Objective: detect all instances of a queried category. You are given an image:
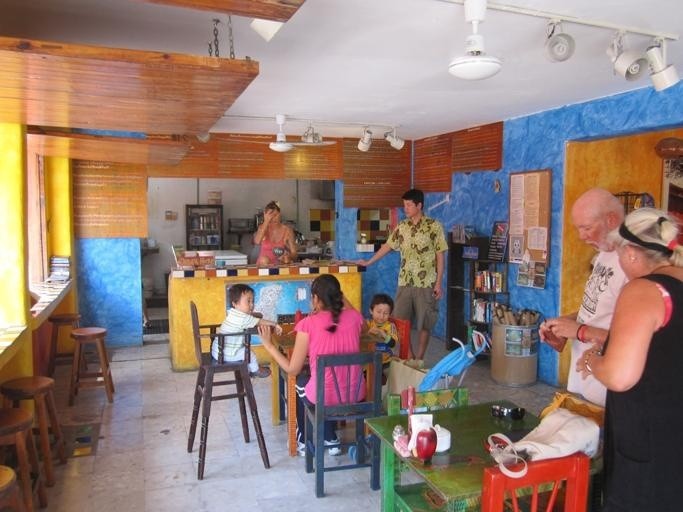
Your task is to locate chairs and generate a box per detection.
[183,298,274,482]
[387,387,472,512]
[277,313,307,421]
[170,244,184,268]
[302,350,384,498]
[481,450,590,512]
[381,313,410,359]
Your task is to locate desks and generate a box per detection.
[192,249,248,266]
[264,320,387,458]
[360,394,607,512]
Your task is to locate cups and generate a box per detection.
[216,261,225,268]
[489,405,525,420]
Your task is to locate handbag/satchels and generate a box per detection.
[541,389,605,428]
[487,407,601,477]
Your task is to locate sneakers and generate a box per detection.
[288,441,306,456]
[324,435,343,455]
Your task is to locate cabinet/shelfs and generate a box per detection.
[184,202,224,250]
[474,258,508,357]
[444,232,487,361]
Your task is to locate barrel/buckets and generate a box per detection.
[490,318,540,389]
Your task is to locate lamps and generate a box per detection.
[603,30,643,79]
[355,125,378,152]
[643,33,679,92]
[382,126,408,150]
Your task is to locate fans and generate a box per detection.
[438,0,500,81]
[220,114,337,153]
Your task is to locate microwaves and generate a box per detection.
[229,218,254,233]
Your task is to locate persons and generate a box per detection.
[355,188,449,360]
[286,219,305,246]
[364,293,400,378]
[211,283,282,378]
[253,201,297,266]
[257,273,365,457]
[538,188,628,408]
[576,207,683,512]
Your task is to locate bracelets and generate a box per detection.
[583,348,602,375]
[577,323,586,342]
[580,325,588,344]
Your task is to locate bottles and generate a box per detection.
[208,190,222,204]
[361,233,367,244]
[255,207,262,215]
[392,424,451,464]
[475,271,504,293]
[374,238,386,252]
[273,247,290,265]
[190,211,218,245]
[284,220,333,257]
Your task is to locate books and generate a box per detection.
[46,254,72,284]
[452,223,475,244]
[472,271,505,354]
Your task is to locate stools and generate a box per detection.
[0,375,68,487]
[0,464,24,512]
[66,327,115,406]
[0,407,50,511]
[43,312,91,380]
